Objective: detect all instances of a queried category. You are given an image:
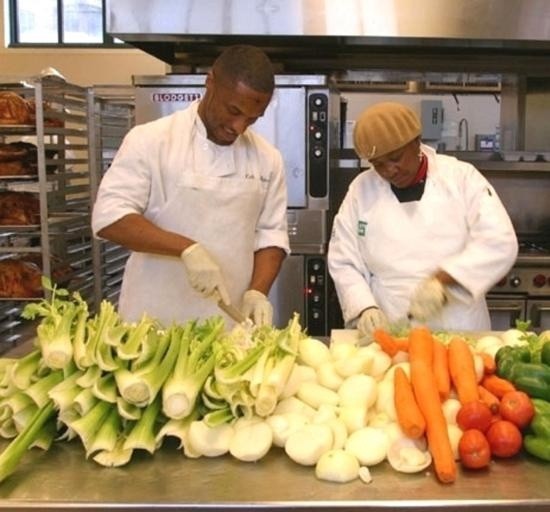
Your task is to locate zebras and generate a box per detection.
[129,73,343,335]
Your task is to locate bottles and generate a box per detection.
[352,102,423,161]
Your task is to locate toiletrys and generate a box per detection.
[495,342,550,461]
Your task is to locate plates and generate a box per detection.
[0,80,135,348]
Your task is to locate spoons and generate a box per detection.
[456,392,535,469]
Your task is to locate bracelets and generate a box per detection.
[447,150,550,161]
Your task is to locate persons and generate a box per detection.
[326,101,519,337]
[90,45,293,329]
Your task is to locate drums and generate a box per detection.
[210,289,246,324]
[354,294,447,348]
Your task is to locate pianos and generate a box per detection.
[372,327,516,485]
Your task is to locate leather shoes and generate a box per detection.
[240,288,275,333]
[408,278,450,323]
[356,302,392,342]
[180,243,230,310]
